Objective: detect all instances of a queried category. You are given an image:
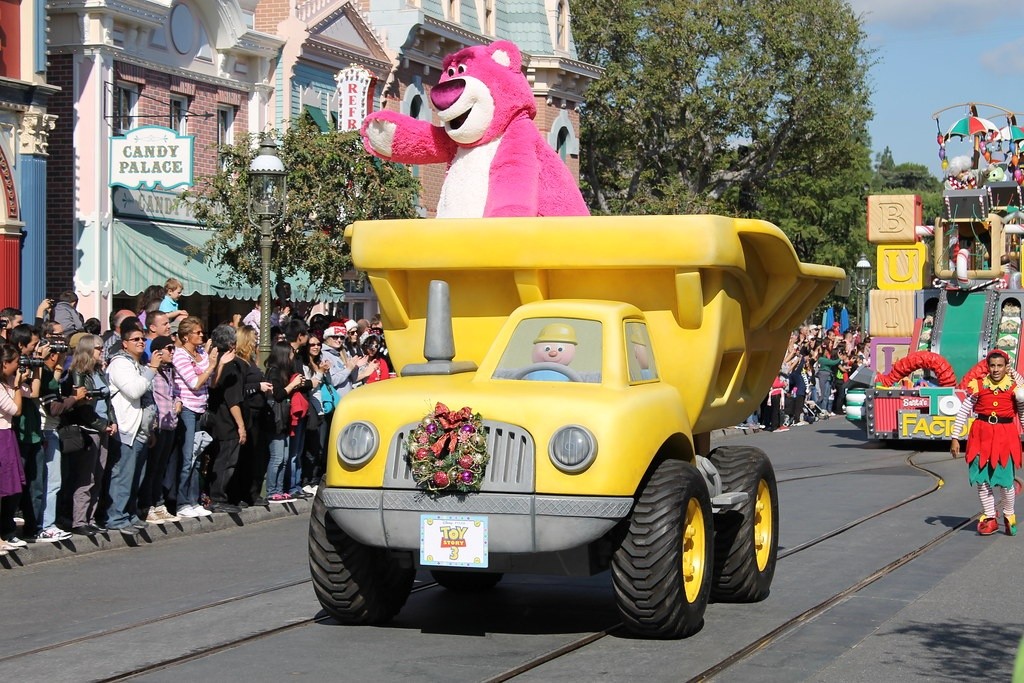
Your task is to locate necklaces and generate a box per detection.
[182,345,197,358]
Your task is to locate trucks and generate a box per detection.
[307,213,845,641]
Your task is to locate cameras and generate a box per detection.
[39,340,68,353]
[158,350,163,356]
[48,301,57,308]
[83,388,101,398]
[18,355,45,373]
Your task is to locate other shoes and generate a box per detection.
[0,541,19,556]
[980,518,999,535]
[1003,514,1017,536]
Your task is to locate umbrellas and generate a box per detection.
[998,125,1024,142]
[943,117,999,142]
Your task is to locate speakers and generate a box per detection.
[867,195,930,381]
[942,181,1019,219]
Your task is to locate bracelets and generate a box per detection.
[150,365,159,368]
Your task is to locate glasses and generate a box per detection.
[163,345,175,352]
[309,343,321,347]
[348,330,358,335]
[94,346,103,351]
[365,344,379,348]
[328,335,345,341]
[125,337,147,341]
[189,330,204,335]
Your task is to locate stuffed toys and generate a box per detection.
[983,162,1009,182]
[361,39,591,218]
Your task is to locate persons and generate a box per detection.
[733,322,872,433]
[135,285,165,329]
[142,311,277,523]
[0,298,164,556]
[44,291,83,336]
[243,294,398,503]
[951,349,1024,535]
[159,278,189,318]
[942,156,991,267]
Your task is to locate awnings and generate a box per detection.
[114,224,348,304]
[304,104,333,132]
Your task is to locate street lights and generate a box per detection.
[852,252,874,342]
[243,133,291,375]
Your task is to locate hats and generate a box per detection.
[341,317,358,332]
[150,336,176,354]
[359,331,380,350]
[323,319,347,340]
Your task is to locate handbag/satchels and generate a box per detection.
[320,376,341,415]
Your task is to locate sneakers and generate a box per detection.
[735,422,750,430]
[5,536,28,547]
[46,526,73,540]
[772,425,790,433]
[73,482,318,537]
[22,529,59,543]
